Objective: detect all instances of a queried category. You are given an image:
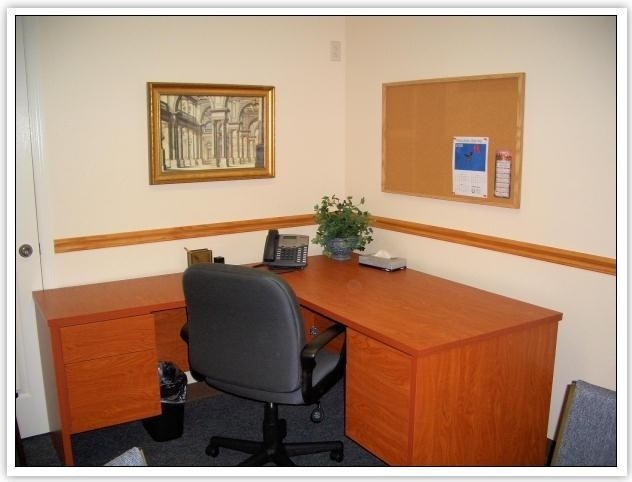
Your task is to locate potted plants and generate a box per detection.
[310,192,376,260]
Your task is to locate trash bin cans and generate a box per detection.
[141,361,187,443]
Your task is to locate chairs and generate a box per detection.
[179,261,346,468]
[550,379,617,467]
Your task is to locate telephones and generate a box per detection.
[263,229,309,270]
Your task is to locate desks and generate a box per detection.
[31,252,563,467]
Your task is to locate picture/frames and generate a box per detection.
[148,81,275,185]
